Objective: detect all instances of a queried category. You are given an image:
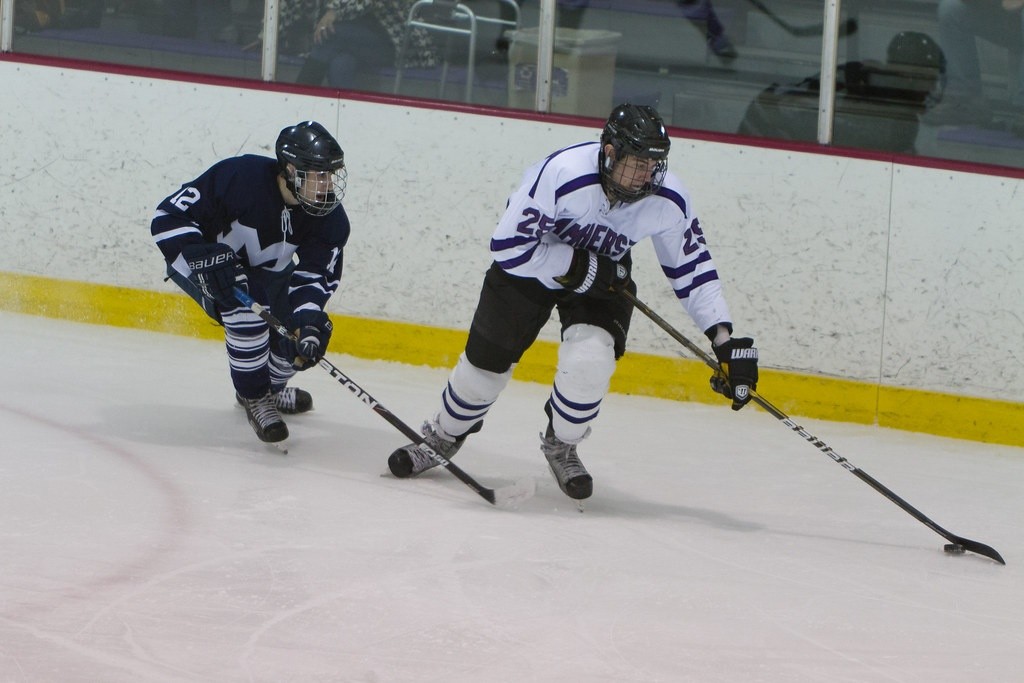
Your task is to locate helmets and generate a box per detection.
[275,121,347,216]
[599,103,671,203]
[886,31,947,72]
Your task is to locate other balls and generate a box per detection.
[943,543,966,554]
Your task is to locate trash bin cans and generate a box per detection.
[504,26,623,122]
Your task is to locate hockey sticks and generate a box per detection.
[234,282,558,513]
[620,283,1005,565]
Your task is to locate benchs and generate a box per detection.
[11,0,1024,168]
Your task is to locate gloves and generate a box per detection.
[184,244,248,308]
[710,337,759,411]
[280,309,333,371]
[552,248,630,301]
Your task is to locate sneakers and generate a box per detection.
[234,386,314,417]
[706,35,737,63]
[242,391,289,454]
[388,411,483,479]
[926,87,992,124]
[539,427,593,513]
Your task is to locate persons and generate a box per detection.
[12,0,438,93]
[147,119,351,444]
[391,104,761,499]
[546,1,949,152]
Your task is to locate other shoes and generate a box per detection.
[216,25,240,44]
